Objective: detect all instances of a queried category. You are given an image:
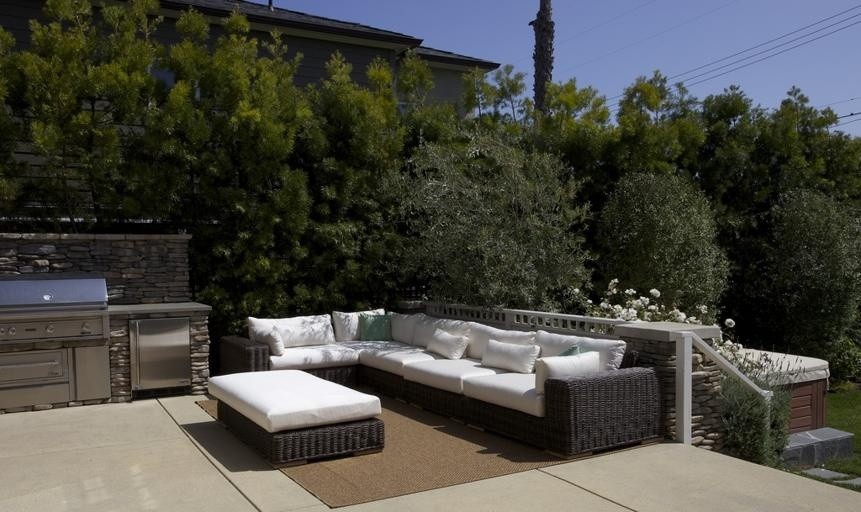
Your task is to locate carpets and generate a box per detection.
[194,384,665,509]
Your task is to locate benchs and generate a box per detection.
[206,369,385,468]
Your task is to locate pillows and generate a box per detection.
[579,335,627,371]
[413,314,470,357]
[248,327,286,357]
[533,350,602,395]
[531,331,581,376]
[332,308,385,342]
[385,311,425,347]
[359,314,392,342]
[426,327,470,360]
[479,338,540,375]
[467,321,536,363]
[558,344,581,357]
[246,313,337,348]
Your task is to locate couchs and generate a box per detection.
[220,308,666,460]
